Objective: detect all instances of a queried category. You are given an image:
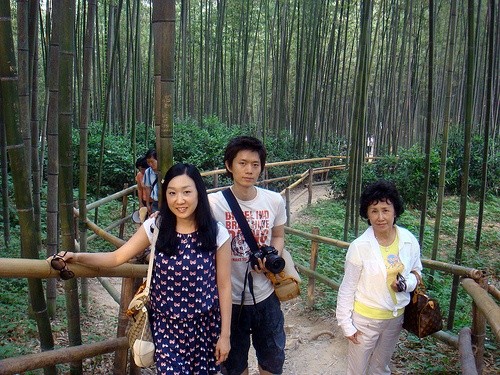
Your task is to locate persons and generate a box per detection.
[336,179,424,375]
[47,164,232,375]
[150,136,288,375]
[136,148,158,222]
[367,134,373,152]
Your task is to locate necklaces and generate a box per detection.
[375,234,393,252]
[178,220,193,241]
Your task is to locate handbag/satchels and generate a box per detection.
[265,248,301,301]
[125,280,155,368]
[401,270,443,338]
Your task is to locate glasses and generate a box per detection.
[51,250,75,280]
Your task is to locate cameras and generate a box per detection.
[251,246,285,274]
